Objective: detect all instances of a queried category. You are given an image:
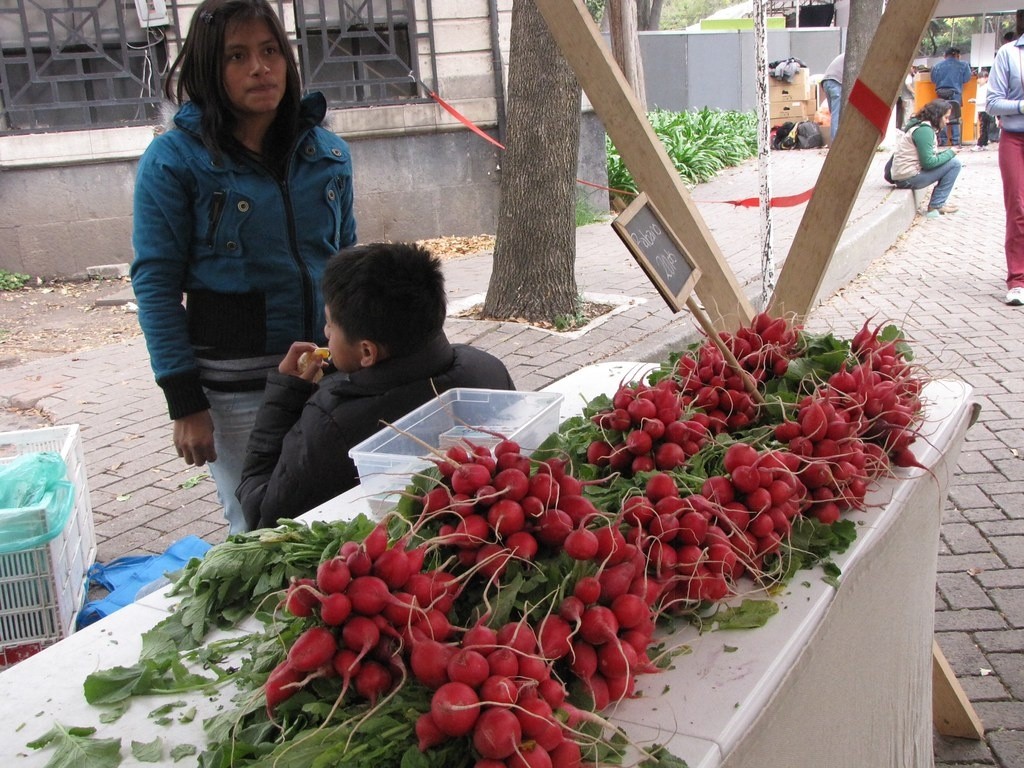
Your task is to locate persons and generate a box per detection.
[820,52,845,145]
[127,1,359,537]
[890,98,962,213]
[900,63,917,130]
[233,241,517,532]
[985,9,1024,306]
[970,31,1017,152]
[930,47,972,146]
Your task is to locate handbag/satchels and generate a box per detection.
[884,154,896,184]
[75,535,215,628]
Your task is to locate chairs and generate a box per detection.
[944,100,964,147]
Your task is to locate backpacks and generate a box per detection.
[770,121,822,150]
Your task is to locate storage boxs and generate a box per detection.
[0,423,98,653]
[768,67,818,129]
[349,388,564,522]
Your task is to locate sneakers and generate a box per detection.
[1005,286,1024,305]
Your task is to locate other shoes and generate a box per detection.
[970,144,988,152]
[928,202,959,214]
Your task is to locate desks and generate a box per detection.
[0,362,972,768]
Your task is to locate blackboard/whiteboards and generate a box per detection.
[612,191,702,315]
